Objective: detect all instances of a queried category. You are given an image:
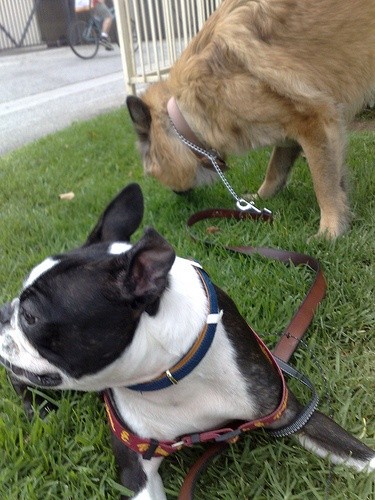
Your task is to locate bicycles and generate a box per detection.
[66,4,145,61]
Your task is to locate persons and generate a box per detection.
[93,0,113,51]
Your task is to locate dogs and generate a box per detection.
[0,181,375,499]
[124,0,375,244]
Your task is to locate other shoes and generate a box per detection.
[98,34,113,51]
